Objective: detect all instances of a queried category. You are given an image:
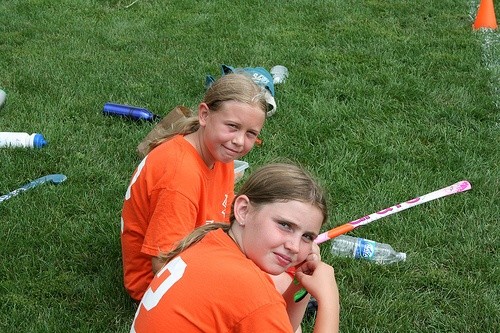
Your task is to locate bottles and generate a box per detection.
[332,234,406,266]
[0,132,47,149]
[103,103,156,121]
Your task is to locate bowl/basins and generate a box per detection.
[233,160,249,185]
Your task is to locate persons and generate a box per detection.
[130,162,340,333]
[120,73,318,314]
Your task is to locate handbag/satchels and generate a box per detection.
[136,106,200,161]
